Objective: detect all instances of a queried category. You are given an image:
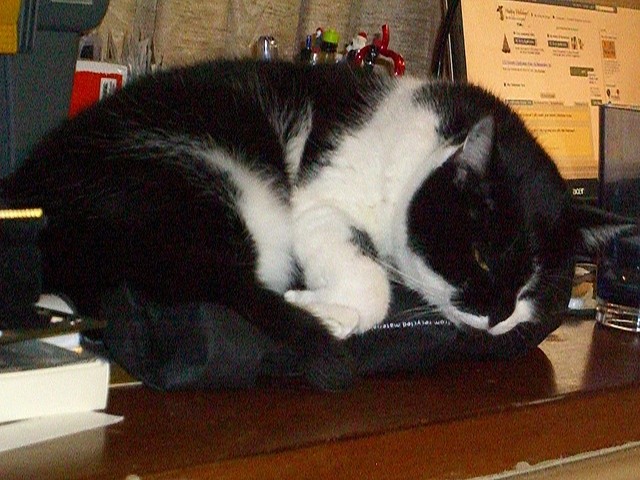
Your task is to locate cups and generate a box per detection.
[567,262,597,309]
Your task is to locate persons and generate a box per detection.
[0,340,112,424]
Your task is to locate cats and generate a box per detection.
[0,52,640,395]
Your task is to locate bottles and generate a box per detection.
[257,37,278,62]
[316,28,338,65]
[301,36,313,65]
